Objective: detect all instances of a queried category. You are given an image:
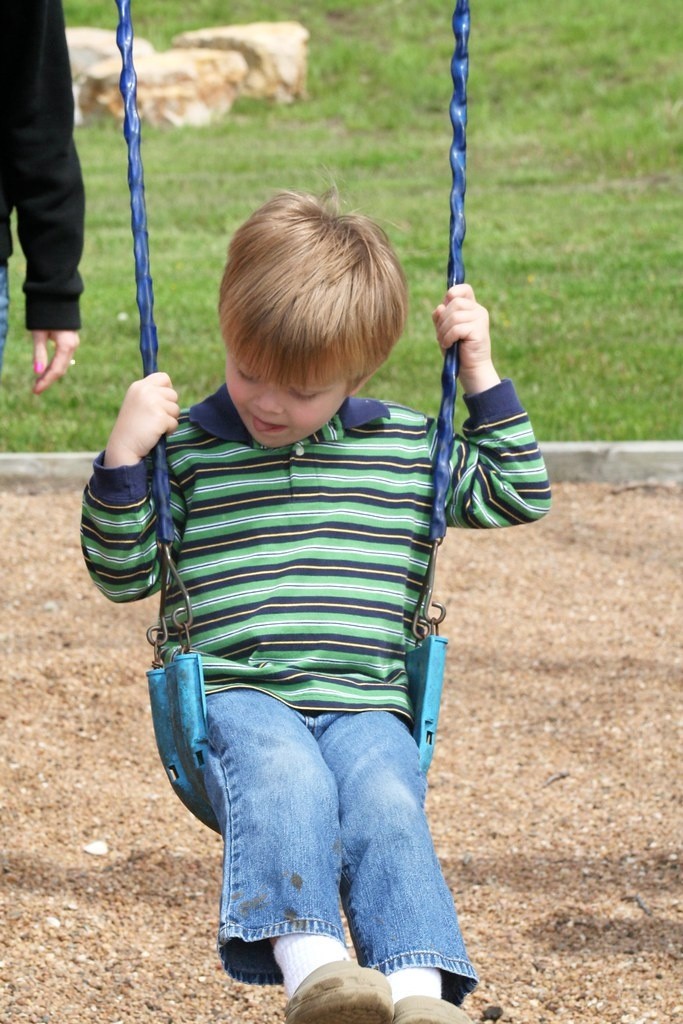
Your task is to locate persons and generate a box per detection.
[83,193,550,1024]
[0,0,87,394]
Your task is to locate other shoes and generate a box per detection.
[282,960,395,1024]
[389,994,472,1024]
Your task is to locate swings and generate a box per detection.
[112,1,477,830]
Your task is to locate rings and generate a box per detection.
[68,359,75,365]
